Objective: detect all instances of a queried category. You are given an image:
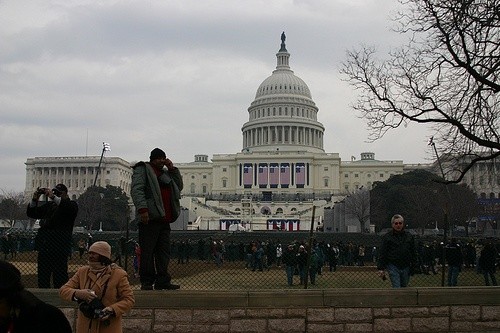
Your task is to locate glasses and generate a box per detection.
[395,222,402,225]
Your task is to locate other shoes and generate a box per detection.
[141,282,153,290]
[154,283,180,289]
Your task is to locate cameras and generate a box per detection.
[94,309,106,319]
[38,188,47,194]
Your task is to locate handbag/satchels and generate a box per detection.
[79,299,103,319]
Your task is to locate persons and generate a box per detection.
[0,213,500,288]
[26,183,78,289]
[0,260,73,333]
[130,148,183,290]
[59,241,135,333]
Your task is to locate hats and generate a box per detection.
[52,183,66,192]
[88,241,111,259]
[149,148,166,162]
